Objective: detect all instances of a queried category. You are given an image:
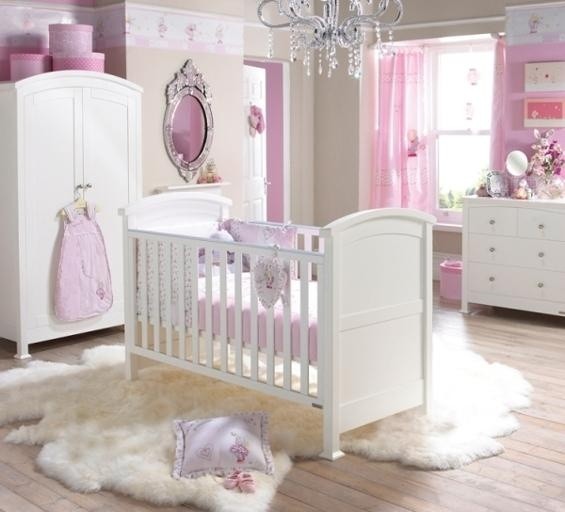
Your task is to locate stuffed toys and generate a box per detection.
[531,128,556,160]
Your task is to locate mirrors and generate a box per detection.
[504,149,529,181]
[163,59,214,182]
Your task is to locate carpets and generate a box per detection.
[1,344,534,512]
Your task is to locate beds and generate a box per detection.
[117,192,437,462]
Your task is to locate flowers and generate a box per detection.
[526,129,564,175]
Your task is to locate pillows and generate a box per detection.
[172,411,276,480]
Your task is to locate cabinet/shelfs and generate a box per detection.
[0,69,144,360]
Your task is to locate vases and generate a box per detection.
[536,174,564,200]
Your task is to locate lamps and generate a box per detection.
[257,1,403,78]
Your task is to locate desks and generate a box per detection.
[460,195,565,317]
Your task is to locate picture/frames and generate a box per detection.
[524,98,565,128]
